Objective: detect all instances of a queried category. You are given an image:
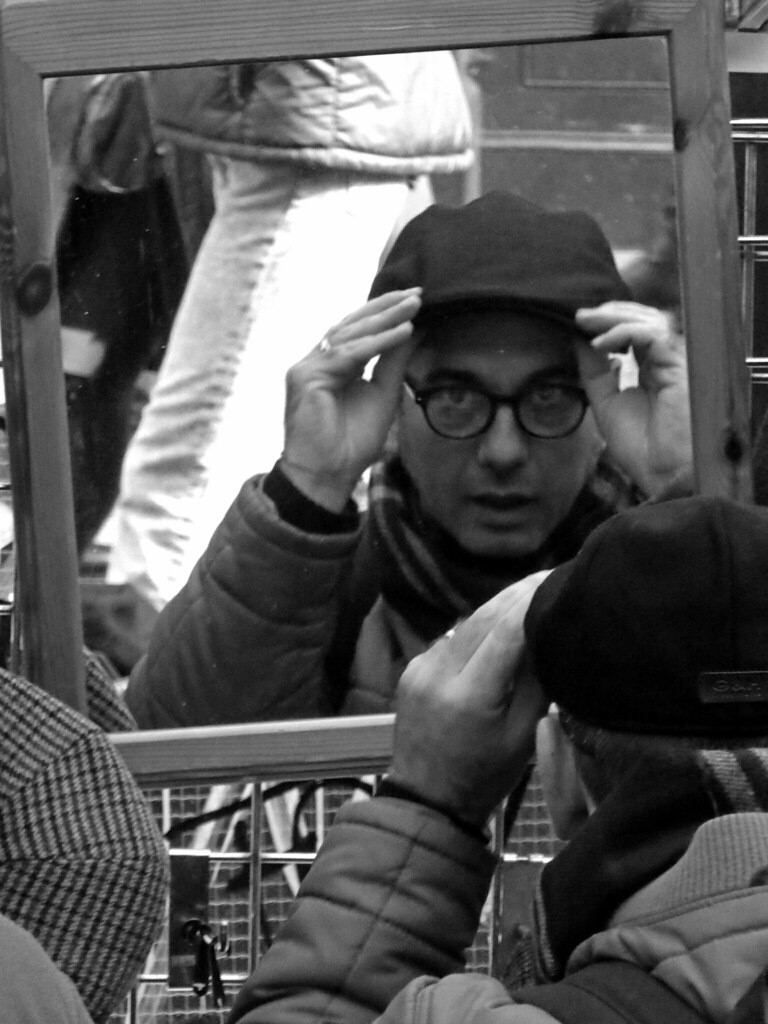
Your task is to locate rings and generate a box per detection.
[317,338,332,354]
[445,628,456,640]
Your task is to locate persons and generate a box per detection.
[129,193,699,730]
[231,493,767,1024]
[39,46,479,619]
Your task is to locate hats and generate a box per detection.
[367,190,635,355]
[523,496,768,736]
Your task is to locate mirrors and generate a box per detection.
[0,0,751,778]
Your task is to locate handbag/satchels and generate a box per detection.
[55,76,196,350]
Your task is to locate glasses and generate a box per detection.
[402,376,590,437]
